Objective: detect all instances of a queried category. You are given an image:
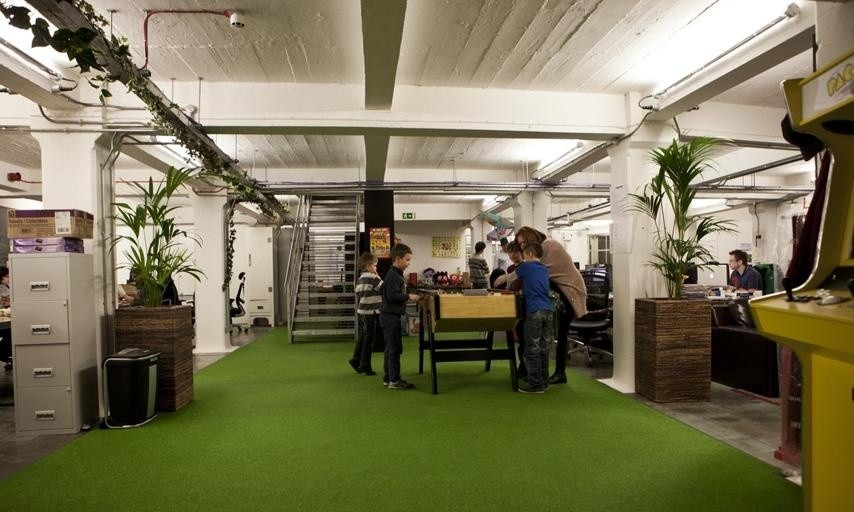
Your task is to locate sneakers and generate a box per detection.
[515,367,550,395]
[349,358,362,374]
[550,372,568,385]
[359,364,376,376]
[382,378,415,391]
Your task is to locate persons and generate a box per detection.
[379,244,420,388]
[0,265,10,309]
[348,252,383,374]
[467,228,588,393]
[117,264,178,306]
[727,250,764,301]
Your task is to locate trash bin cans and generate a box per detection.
[101,348,162,429]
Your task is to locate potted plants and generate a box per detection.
[627,135,738,404]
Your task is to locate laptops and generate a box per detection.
[697,265,727,286]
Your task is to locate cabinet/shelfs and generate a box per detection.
[8,252,101,438]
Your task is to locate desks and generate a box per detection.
[679,282,751,328]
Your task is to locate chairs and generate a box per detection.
[231,282,248,335]
[566,272,612,368]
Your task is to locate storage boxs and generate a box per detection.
[406,312,419,337]
[8,239,84,246]
[100,165,198,413]
[8,210,95,239]
[9,246,85,253]
